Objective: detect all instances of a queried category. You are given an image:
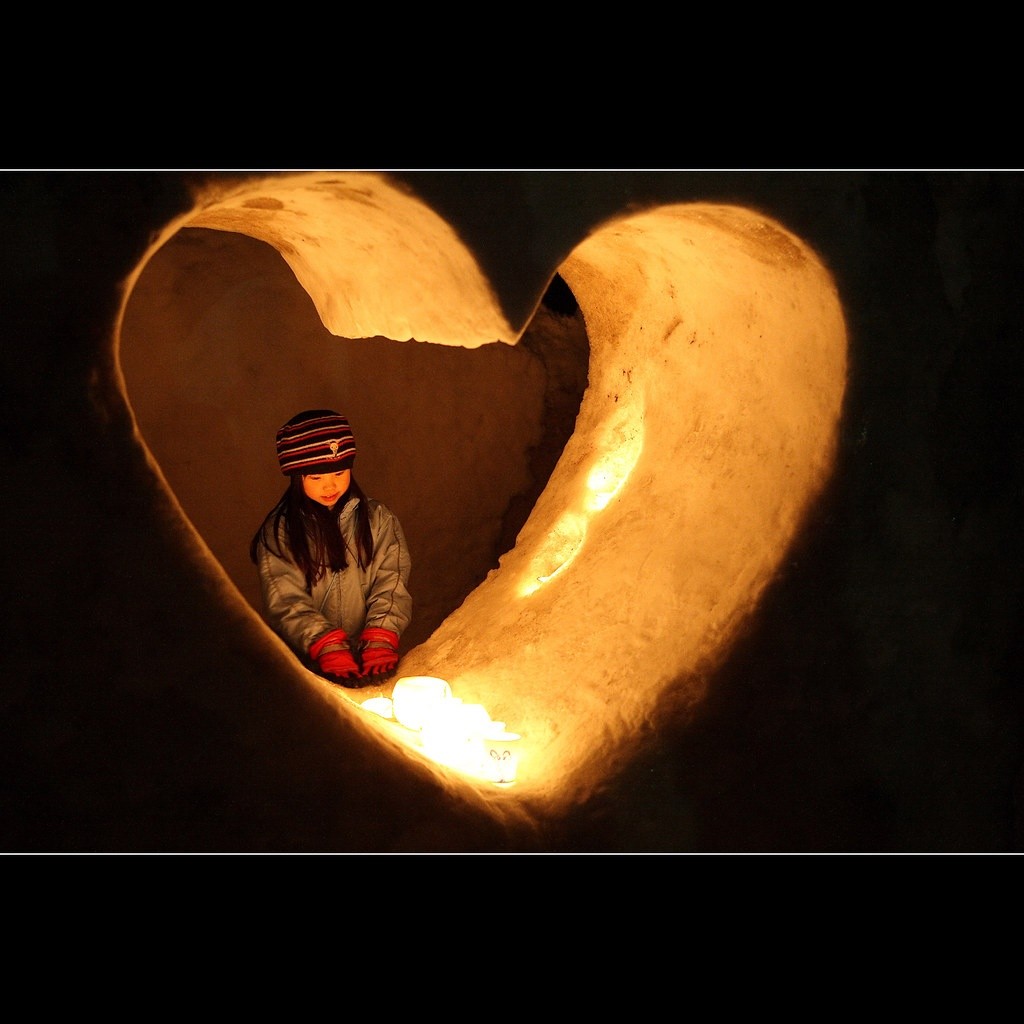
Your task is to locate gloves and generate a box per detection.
[309,628,365,689]
[355,627,399,685]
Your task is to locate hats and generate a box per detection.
[276,410,356,476]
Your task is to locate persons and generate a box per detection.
[248,408,413,688]
